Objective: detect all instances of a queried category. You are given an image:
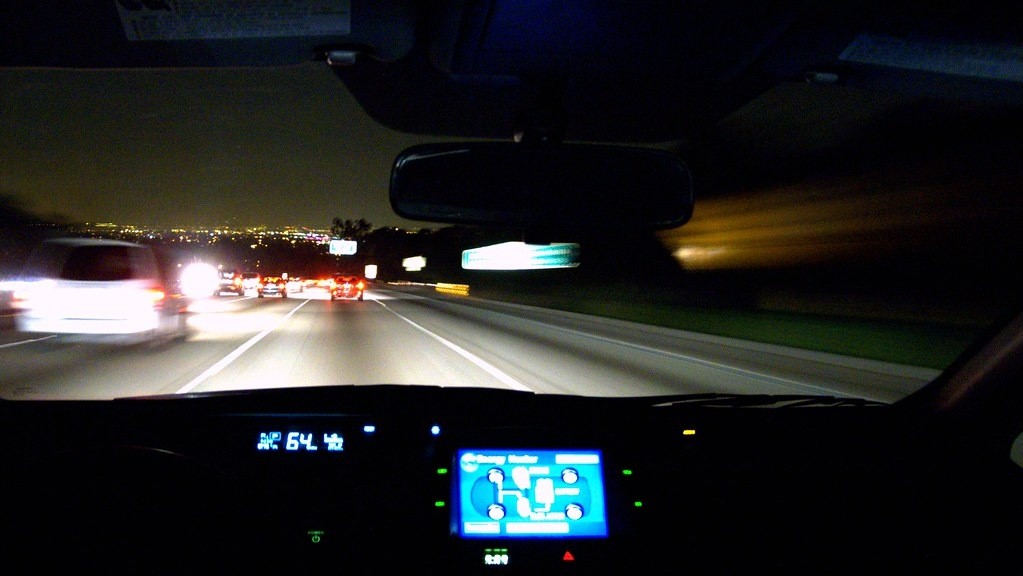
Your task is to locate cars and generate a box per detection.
[331,276,364,301]
[214,270,333,297]
[10,235,191,347]
